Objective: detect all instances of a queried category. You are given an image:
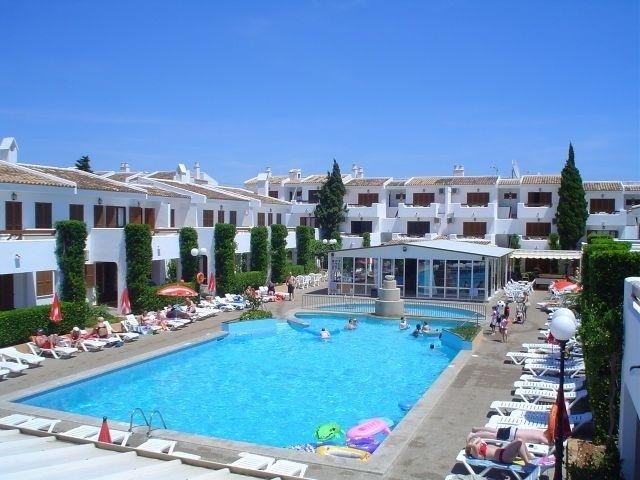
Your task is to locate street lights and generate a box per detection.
[322,238,338,253]
[549,308,577,480]
[190,247,207,306]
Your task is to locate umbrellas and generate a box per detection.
[48,292,64,325]
[541,392,574,446]
[120,288,132,315]
[208,273,215,303]
[157,285,198,320]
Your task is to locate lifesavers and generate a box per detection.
[344,438,380,453]
[197,272,204,285]
[315,418,390,441]
[316,445,371,460]
[548,400,571,445]
[422,327,435,335]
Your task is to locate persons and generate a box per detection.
[464,437,538,469]
[69,327,82,351]
[135,298,197,332]
[320,327,330,339]
[251,288,258,298]
[353,318,360,327]
[521,288,529,321]
[344,319,355,331]
[247,285,252,301]
[490,300,509,342]
[546,332,554,345]
[515,299,525,323]
[285,271,297,302]
[86,316,108,339]
[400,316,410,330]
[463,425,557,446]
[266,280,275,296]
[463,281,470,292]
[35,329,58,350]
[477,281,485,293]
[409,321,442,349]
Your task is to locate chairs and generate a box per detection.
[229,455,308,479]
[1,414,61,434]
[0,269,327,381]
[135,438,202,460]
[59,426,131,448]
[443,278,594,480]
